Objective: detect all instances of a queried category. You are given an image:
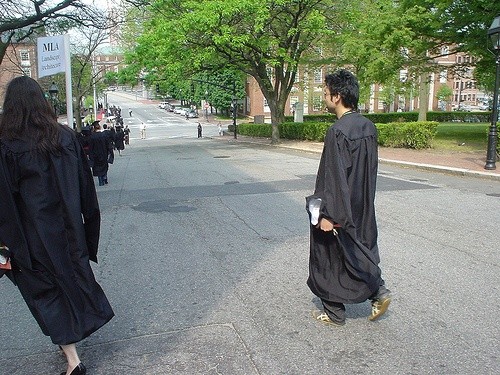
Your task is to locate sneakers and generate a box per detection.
[369,291,392,323]
[310,308,344,326]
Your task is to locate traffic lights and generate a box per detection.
[156,83,159,93]
[191,84,194,93]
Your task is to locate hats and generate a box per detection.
[80,126,89,132]
[91,120,99,127]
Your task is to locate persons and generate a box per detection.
[72,100,132,186]
[140,122,146,138]
[0,76,115,375]
[197,122,203,138]
[218,121,224,135]
[304,69,393,326]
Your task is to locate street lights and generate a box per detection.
[89,104,93,122]
[484,15,500,170]
[232,95,240,139]
[48,81,59,123]
[204,90,210,123]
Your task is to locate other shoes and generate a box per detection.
[99,179,108,186]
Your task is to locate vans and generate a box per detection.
[107,86,117,91]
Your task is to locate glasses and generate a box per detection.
[324,91,337,100]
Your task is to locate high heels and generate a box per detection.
[59,363,88,375]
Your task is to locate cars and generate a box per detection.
[181,108,189,116]
[173,107,182,114]
[158,102,175,112]
[453,103,472,113]
[186,109,197,117]
[480,98,500,110]
